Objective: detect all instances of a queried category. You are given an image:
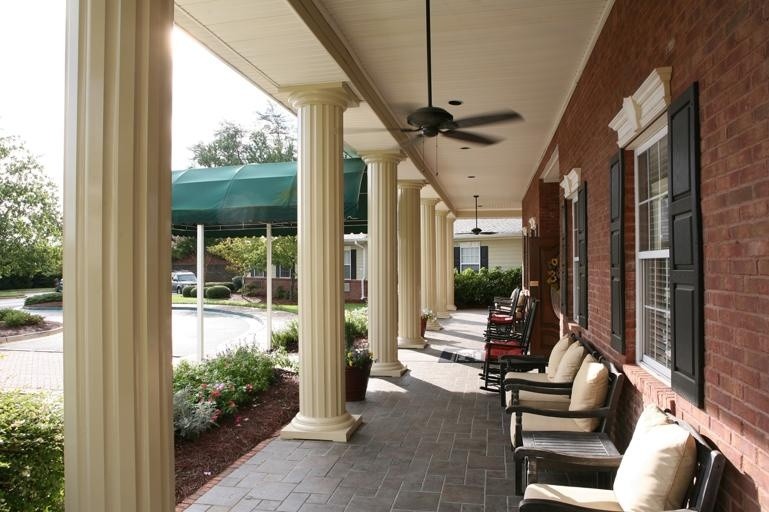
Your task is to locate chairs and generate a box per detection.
[505,357,625,495]
[497,336,600,452]
[497,329,581,407]
[478,286,539,393]
[513,407,725,512]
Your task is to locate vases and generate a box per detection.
[421,316,428,337]
[344,362,373,401]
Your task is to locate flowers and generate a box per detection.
[420,309,433,320]
[345,339,377,366]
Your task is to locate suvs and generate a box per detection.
[171,269,197,293]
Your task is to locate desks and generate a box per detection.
[519,430,622,498]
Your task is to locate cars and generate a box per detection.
[55,276,63,291]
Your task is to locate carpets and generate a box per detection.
[438,347,485,363]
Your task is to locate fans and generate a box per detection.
[455,194,497,235]
[339,0,526,146]
